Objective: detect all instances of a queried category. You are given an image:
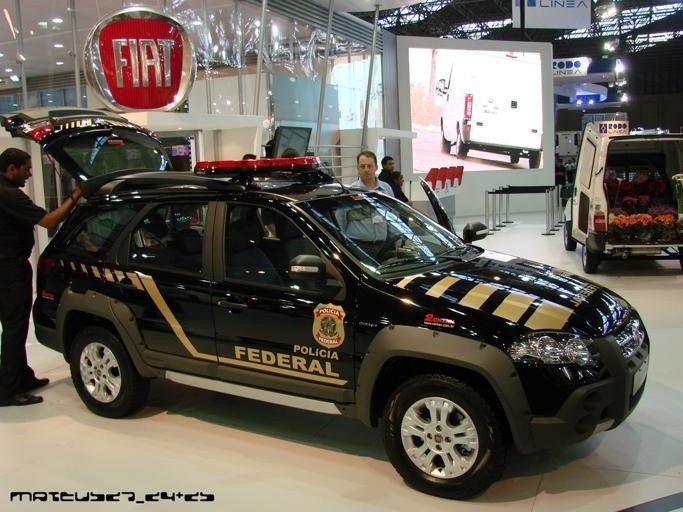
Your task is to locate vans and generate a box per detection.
[558,117,683,275]
[430,50,543,168]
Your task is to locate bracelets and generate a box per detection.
[67,195,75,204]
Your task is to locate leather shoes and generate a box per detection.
[1,378,49,406]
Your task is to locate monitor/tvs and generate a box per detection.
[274,126,312,158]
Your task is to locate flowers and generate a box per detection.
[605,170,683,244]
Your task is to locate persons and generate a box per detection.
[385,170,415,251]
[228,152,259,221]
[332,150,403,261]
[260,148,302,237]
[553,155,578,186]
[376,155,395,186]
[0,146,81,409]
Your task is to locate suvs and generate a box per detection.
[0,107,651,504]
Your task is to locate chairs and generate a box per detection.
[129,216,321,290]
